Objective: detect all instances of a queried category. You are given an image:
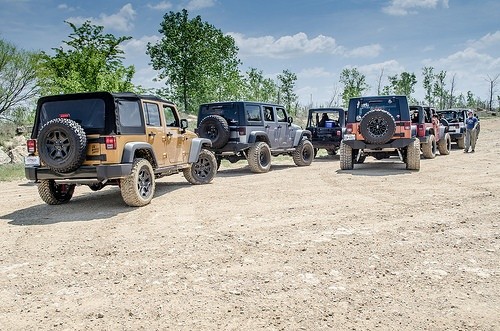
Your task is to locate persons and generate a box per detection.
[463,111,478,153]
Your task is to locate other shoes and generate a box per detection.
[464,149,475,153]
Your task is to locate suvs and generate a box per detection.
[304,107,348,157]
[340,95,421,170]
[24,90,218,209]
[409,105,452,159]
[437,109,480,149]
[195,100,315,173]
